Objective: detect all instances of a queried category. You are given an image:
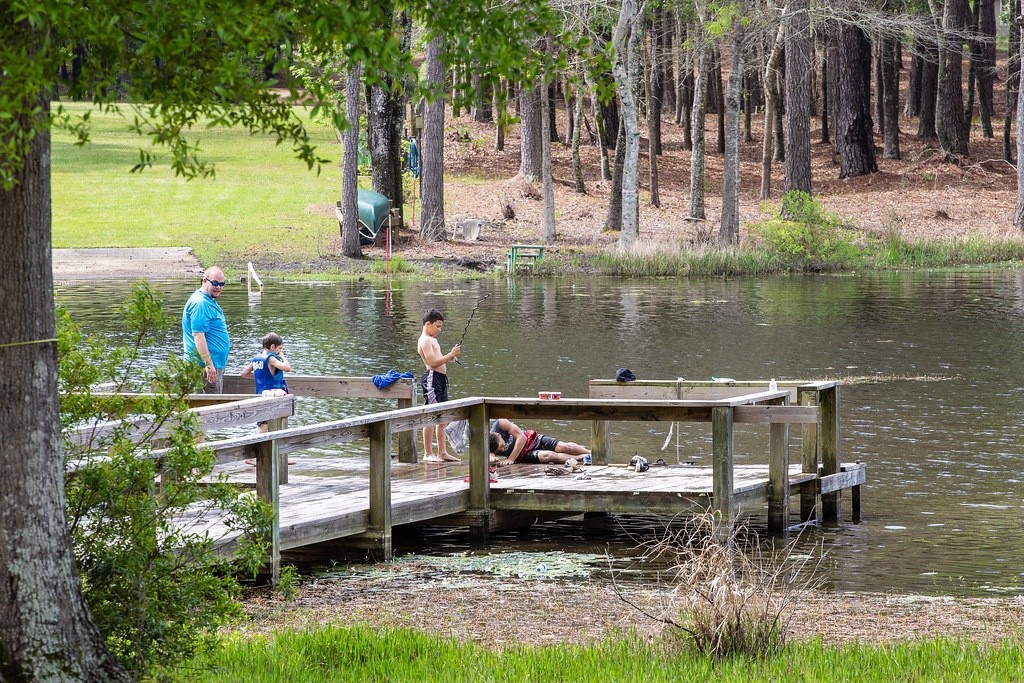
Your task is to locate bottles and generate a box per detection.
[536,562,547,574]
[769,377,778,393]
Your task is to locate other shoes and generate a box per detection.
[635,458,649,472]
[565,458,579,467]
[628,456,648,470]
[583,454,591,465]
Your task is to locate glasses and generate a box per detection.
[205,277,225,287]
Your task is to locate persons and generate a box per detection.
[182,266,230,394]
[417,308,461,462]
[489,419,592,466]
[242,332,297,466]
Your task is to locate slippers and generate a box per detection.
[572,466,586,473]
[652,458,668,465]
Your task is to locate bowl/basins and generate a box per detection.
[550,391,562,400]
[539,391,551,400]
